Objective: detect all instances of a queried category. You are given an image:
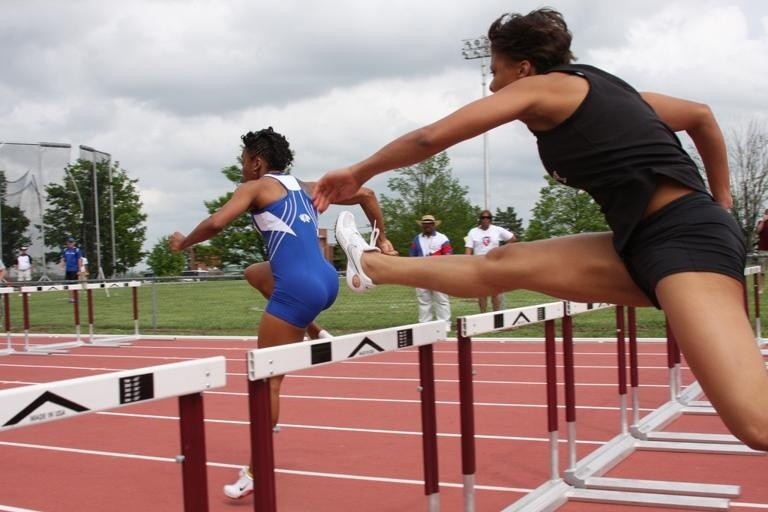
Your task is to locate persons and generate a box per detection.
[165,125,400,501]
[755,208,768,295]
[405,212,456,325]
[15,246,32,297]
[0,259,6,283]
[308,1,768,455]
[461,209,520,309]
[77,255,90,284]
[58,236,83,304]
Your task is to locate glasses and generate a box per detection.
[480,216,490,219]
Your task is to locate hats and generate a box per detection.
[416,214,441,228]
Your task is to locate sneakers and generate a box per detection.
[334,211,380,292]
[223,466,254,499]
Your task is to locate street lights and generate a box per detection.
[460,30,496,225]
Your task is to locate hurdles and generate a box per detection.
[564,266,768,511]
[245,319,446,512]
[0,355,226,512]
[456,301,566,511]
